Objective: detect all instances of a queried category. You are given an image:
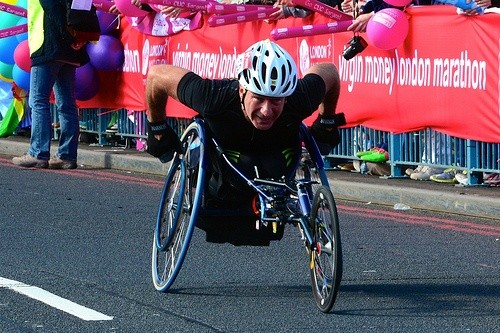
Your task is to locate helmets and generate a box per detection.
[236,39,298,98]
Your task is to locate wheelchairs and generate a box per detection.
[151,116,344,314]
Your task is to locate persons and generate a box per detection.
[17,0,500,186]
[144,39,340,206]
[10,0,96,171]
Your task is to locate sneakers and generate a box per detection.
[48,156,78,170]
[12,153,49,169]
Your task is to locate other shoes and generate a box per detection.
[338,158,500,186]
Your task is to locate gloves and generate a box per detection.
[146,121,187,163]
[311,113,347,156]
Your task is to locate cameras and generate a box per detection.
[342,35,368,61]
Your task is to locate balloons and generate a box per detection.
[112,0,149,18]
[366,8,407,50]
[0,0,125,100]
[382,0,412,7]
